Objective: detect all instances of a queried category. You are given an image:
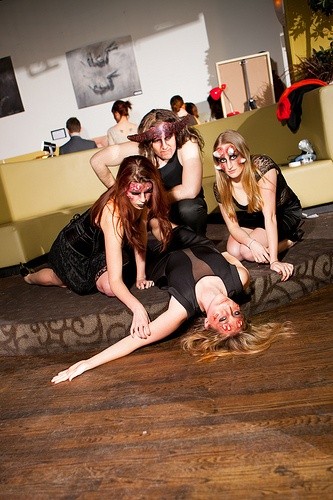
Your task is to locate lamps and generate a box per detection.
[209,83,240,117]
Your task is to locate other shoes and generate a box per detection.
[289,221,306,242]
[19,262,29,276]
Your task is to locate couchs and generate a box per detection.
[0,84,333,278]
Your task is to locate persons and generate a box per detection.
[213,131,303,281]
[59,117,97,155]
[90,109,207,236]
[51,218,249,384]
[170,95,225,125]
[20,156,171,339]
[107,100,138,145]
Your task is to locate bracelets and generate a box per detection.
[271,261,278,266]
[249,239,256,248]
